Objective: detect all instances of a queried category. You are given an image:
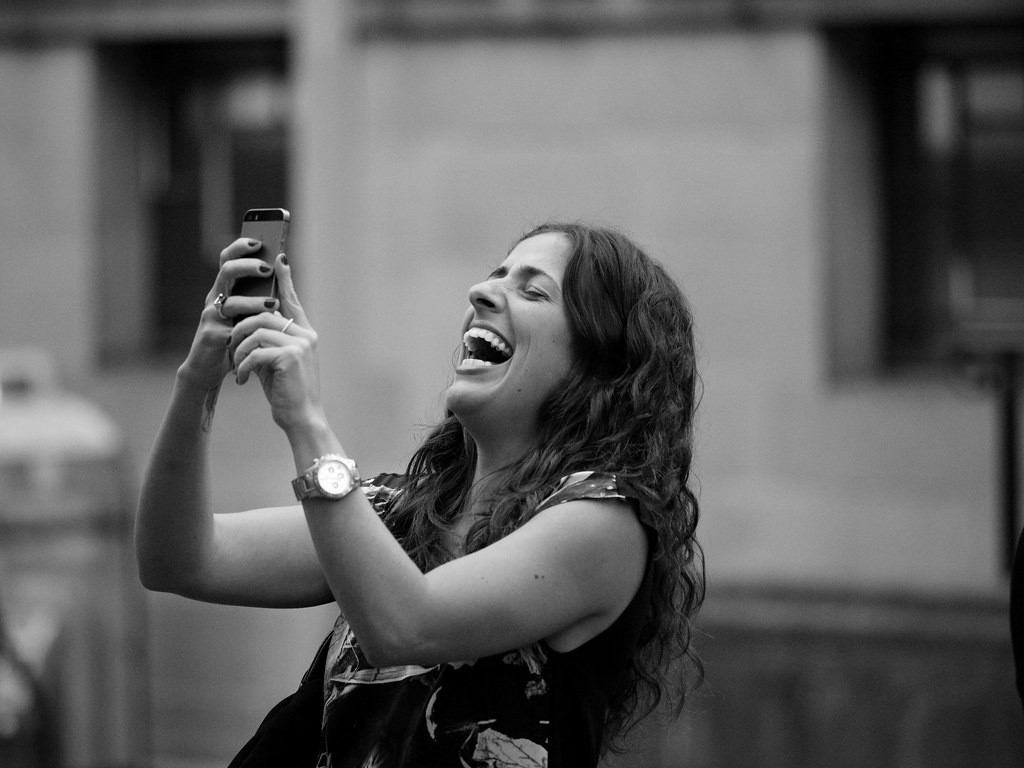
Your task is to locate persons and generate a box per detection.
[137,222,726,768]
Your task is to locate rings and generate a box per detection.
[213,293,231,319]
[282,319,295,333]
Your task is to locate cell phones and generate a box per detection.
[231,209,295,321]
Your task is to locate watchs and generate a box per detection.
[291,452,362,501]
